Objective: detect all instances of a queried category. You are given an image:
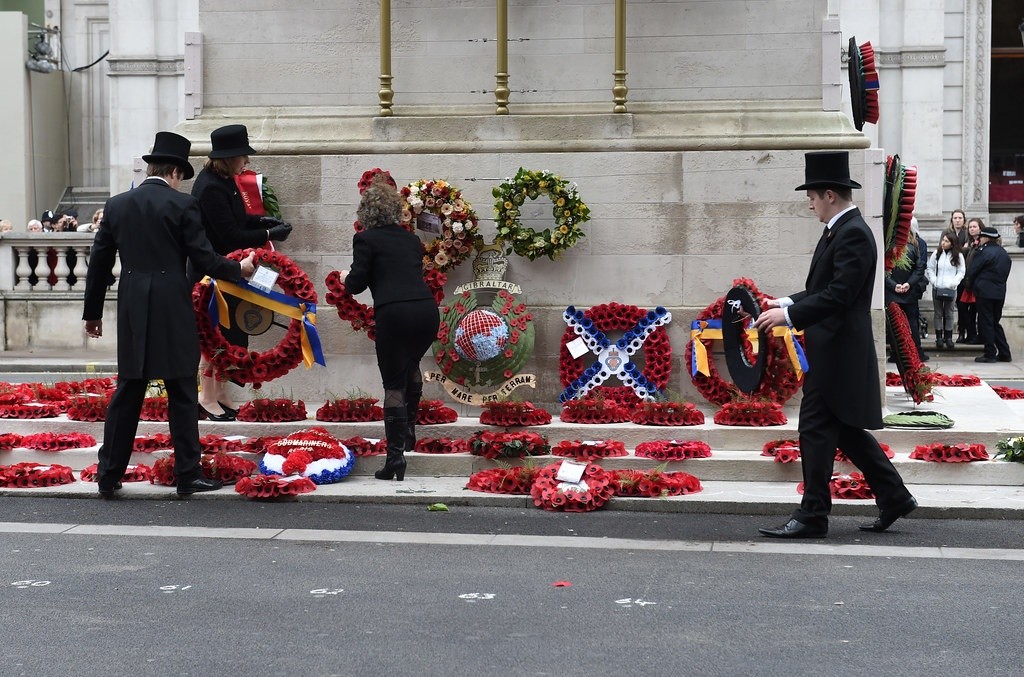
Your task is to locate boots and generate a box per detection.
[944,330,954,348]
[375,406,407,481]
[406,382,423,452]
[934,329,944,348]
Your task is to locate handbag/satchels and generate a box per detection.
[935,288,955,301]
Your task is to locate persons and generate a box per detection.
[0,209,134,289]
[82,131,256,493]
[927,234,965,347]
[340,184,439,480]
[886,213,929,362]
[960,218,984,345]
[968,226,1013,363]
[753,151,917,536]
[940,209,967,344]
[190,124,292,421]
[1014,215,1024,248]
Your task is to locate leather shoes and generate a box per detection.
[859,496,918,531]
[758,518,827,537]
[99,480,123,493]
[177,476,223,494]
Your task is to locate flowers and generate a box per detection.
[884,301,934,405]
[397,178,480,270]
[324,255,446,341]
[560,300,672,402]
[435,290,532,385]
[857,37,880,125]
[492,167,592,262]
[0,371,1024,513]
[190,248,320,390]
[886,154,918,273]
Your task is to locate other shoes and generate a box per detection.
[996,355,1012,362]
[956,330,965,342]
[888,355,895,363]
[917,348,930,361]
[975,354,996,363]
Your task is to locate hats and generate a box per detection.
[795,151,862,190]
[40,211,54,223]
[911,216,920,238]
[67,211,78,218]
[142,132,194,180]
[208,125,256,159]
[978,227,1001,239]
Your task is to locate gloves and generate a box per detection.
[268,223,292,241]
[260,216,284,228]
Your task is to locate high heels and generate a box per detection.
[217,400,240,417]
[197,402,236,421]
